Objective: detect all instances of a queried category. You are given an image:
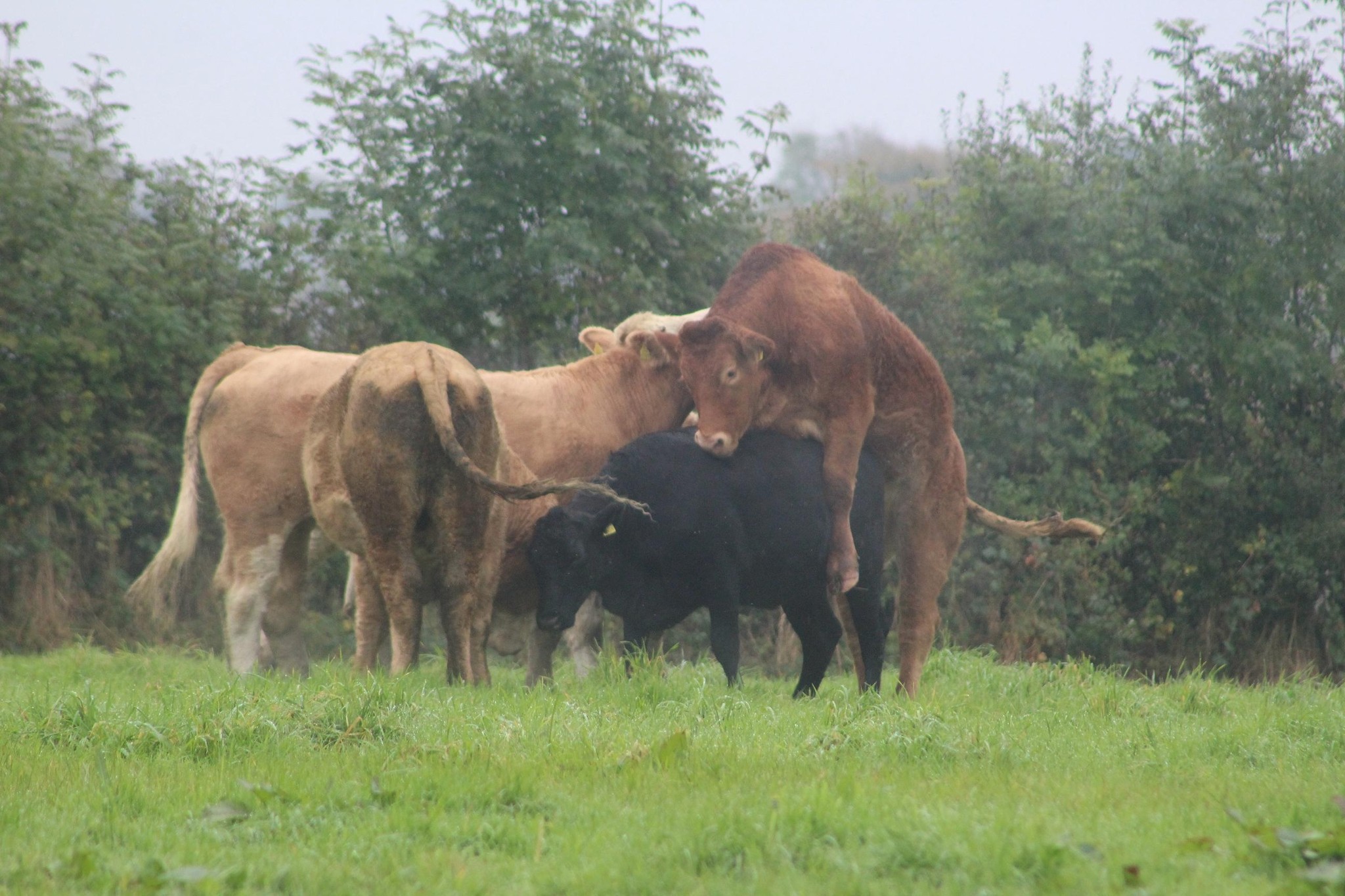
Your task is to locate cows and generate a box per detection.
[298,338,653,684]
[656,244,1104,693]
[126,308,707,673]
[513,425,895,697]
